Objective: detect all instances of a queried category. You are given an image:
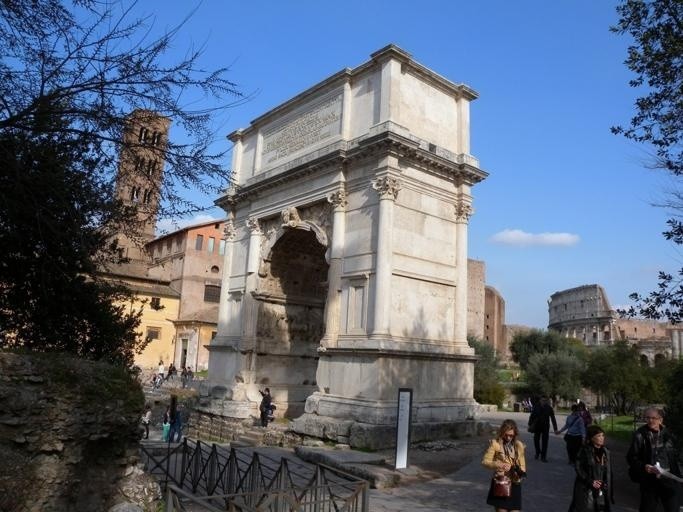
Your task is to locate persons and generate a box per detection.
[259,388,271,428]
[266,405,276,422]
[144,395,183,443]
[482,395,682,512]
[153,360,194,387]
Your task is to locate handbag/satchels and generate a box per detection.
[564,431,570,441]
[528,418,538,433]
[493,475,512,498]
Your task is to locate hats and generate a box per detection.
[588,426,605,440]
